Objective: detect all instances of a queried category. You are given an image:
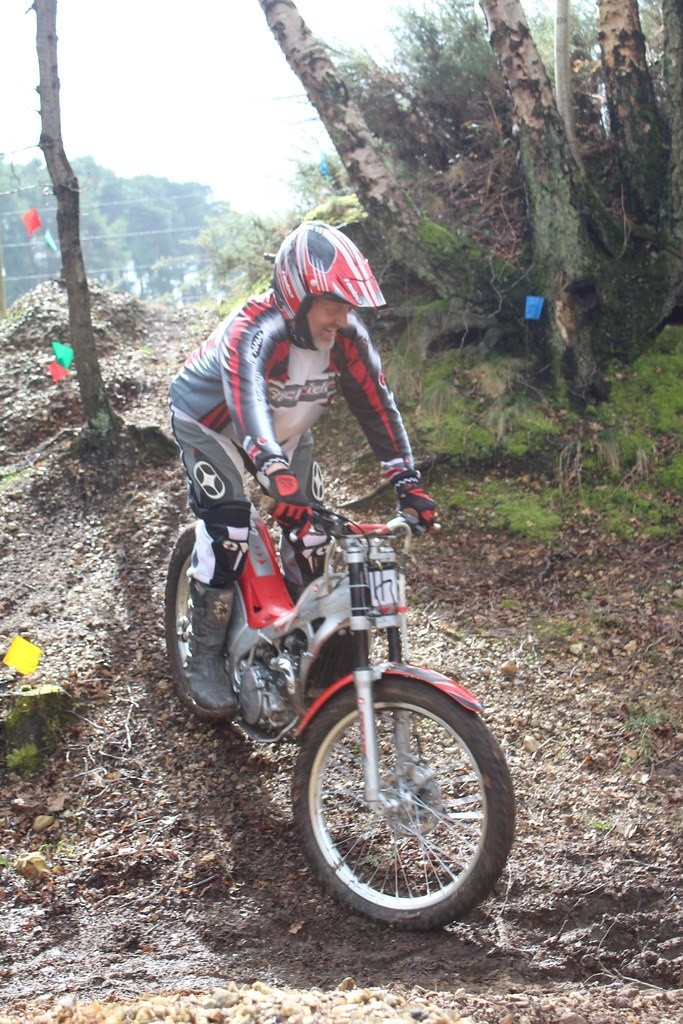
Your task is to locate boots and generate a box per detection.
[177,576,238,717]
[281,576,306,607]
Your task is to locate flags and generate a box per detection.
[2,634,43,677]
[44,224,58,255]
[19,207,41,237]
[46,342,74,384]
[524,296,544,320]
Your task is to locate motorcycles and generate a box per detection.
[163,497,517,933]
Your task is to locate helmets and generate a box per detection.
[273,222,387,321]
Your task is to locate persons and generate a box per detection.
[168,220,442,718]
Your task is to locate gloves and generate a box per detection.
[390,470,435,532]
[269,468,311,539]
